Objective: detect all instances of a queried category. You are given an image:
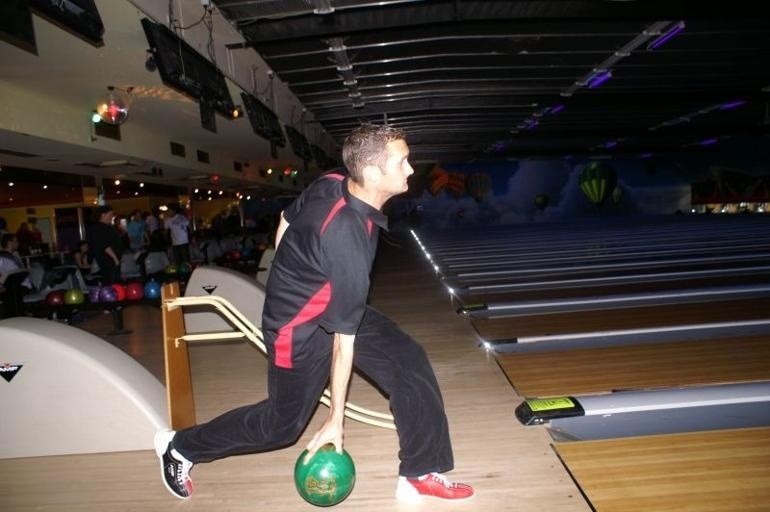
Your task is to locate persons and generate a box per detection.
[0,204,240,293]
[158,124,472,499]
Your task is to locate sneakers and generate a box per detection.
[395,471,474,503]
[154,429,195,499]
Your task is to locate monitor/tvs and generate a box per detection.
[240,91,287,147]
[141,17,238,119]
[285,125,313,161]
[310,143,338,171]
[12,0,108,47]
